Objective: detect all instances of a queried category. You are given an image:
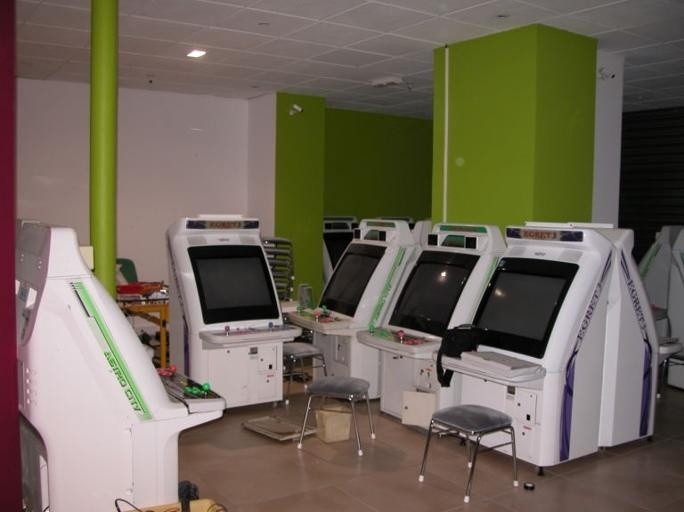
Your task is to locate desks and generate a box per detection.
[115,279,169,371]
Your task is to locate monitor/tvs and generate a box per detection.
[187,244,281,324]
[388,251,481,337]
[323,231,354,272]
[319,243,388,320]
[471,257,580,359]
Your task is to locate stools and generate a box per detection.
[651,307,672,399]
[296,377,377,458]
[416,404,519,505]
[281,342,330,407]
[261,236,295,302]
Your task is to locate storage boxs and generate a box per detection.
[314,402,352,443]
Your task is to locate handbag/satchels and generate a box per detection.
[436,323,478,386]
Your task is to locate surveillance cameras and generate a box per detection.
[292,104,304,113]
[600,67,616,80]
